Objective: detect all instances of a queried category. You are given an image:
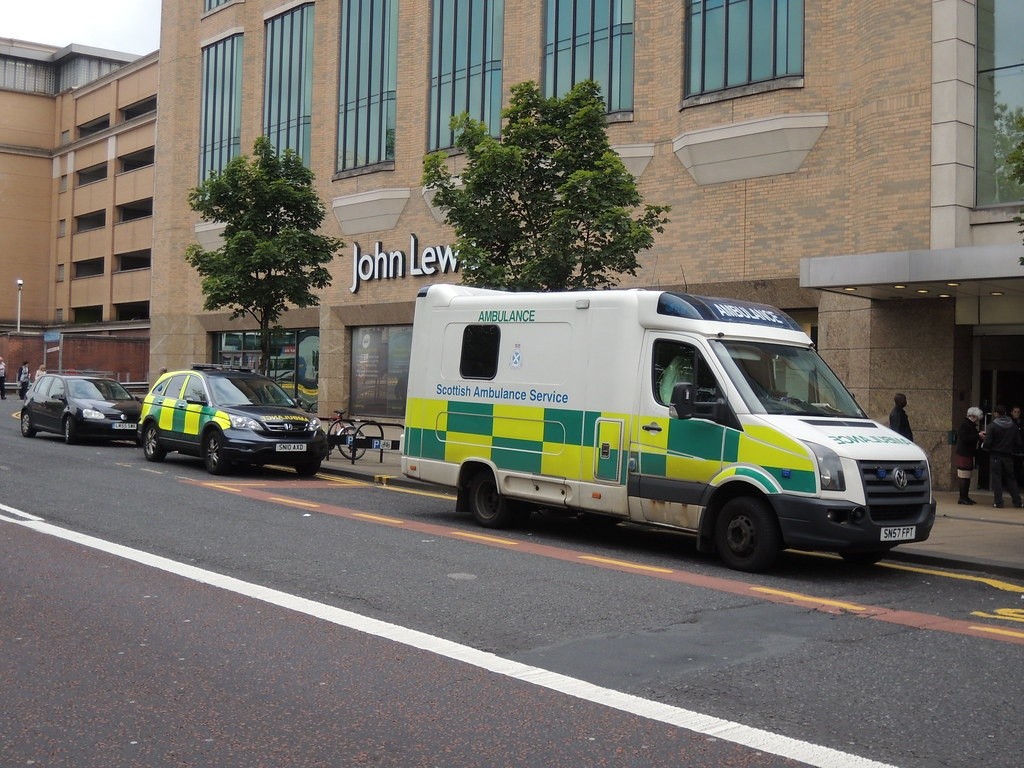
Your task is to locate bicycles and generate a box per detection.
[297,398,367,461]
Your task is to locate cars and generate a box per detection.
[20,373,140,445]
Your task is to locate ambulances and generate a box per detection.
[399,283,937,576]
[136,363,328,476]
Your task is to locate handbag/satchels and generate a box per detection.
[975,438,989,472]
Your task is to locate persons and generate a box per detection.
[954,405,1024,509]
[34,364,46,381]
[17,361,31,400]
[157,367,172,376]
[890,393,914,441]
[0,357,7,400]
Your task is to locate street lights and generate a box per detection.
[16,278,23,332]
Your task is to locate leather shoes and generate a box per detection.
[958,496,976,505]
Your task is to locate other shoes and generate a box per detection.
[1015,502,1024,508]
[993,502,1004,508]
[2,397,6,399]
